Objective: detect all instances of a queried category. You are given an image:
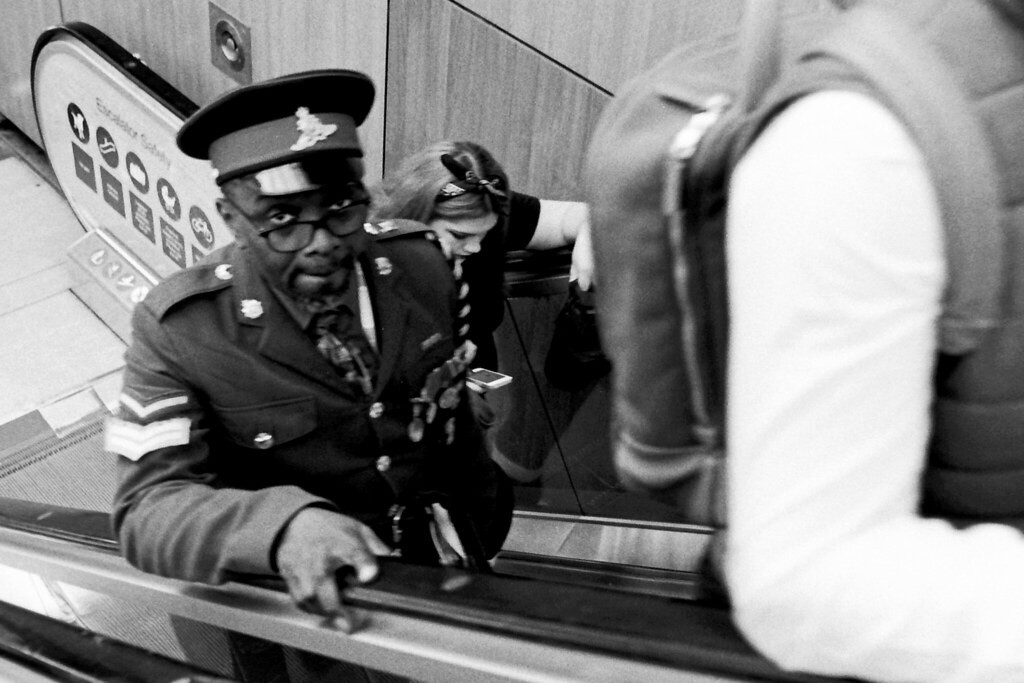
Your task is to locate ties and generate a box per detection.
[314,315,373,396]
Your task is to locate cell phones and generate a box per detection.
[466,368,513,390]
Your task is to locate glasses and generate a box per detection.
[228,188,371,253]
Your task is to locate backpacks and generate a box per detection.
[584,0,1005,496]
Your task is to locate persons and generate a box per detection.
[366,140,599,437]
[716,1,1024,682]
[110,68,516,635]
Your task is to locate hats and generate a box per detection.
[176,68,375,196]
